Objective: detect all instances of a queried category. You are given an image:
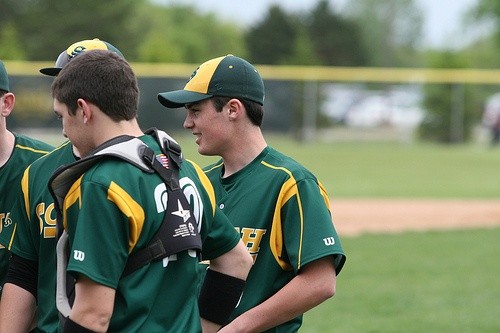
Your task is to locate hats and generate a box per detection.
[157,54,264,109]
[39,38,125,76]
[0,61,10,92]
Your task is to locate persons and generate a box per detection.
[0,40,142,333]
[53,49,253,332]
[158,55,346,333]
[0,60,57,278]
